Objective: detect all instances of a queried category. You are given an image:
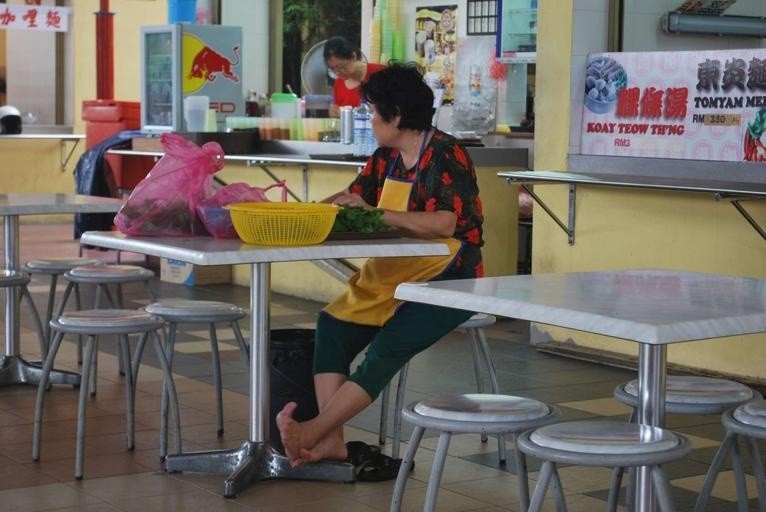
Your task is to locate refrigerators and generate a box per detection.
[139,23,244,135]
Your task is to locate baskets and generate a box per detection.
[225,201,341,246]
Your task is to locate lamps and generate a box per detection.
[662,12,766,40]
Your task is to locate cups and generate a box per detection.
[416,21,437,64]
[183,95,210,132]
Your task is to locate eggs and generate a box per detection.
[586,75,606,100]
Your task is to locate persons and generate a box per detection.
[275,59,485,464]
[324,36,388,117]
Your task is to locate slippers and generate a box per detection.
[346,441,415,483]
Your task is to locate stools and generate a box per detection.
[518,419,690,510]
[694,399,765,510]
[23,257,100,348]
[377,313,508,468]
[132,299,248,461]
[1,267,51,388]
[391,392,566,510]
[31,308,182,478]
[604,378,751,508]
[55,263,156,376]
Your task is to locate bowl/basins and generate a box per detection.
[585,56,628,113]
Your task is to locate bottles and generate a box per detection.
[340,100,373,151]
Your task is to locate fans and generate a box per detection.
[299,40,368,97]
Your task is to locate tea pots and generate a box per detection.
[270,92,299,139]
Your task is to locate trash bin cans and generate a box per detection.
[267,327,316,459]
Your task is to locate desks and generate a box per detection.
[390,268,765,511]
[78,229,451,499]
[1,191,125,390]
